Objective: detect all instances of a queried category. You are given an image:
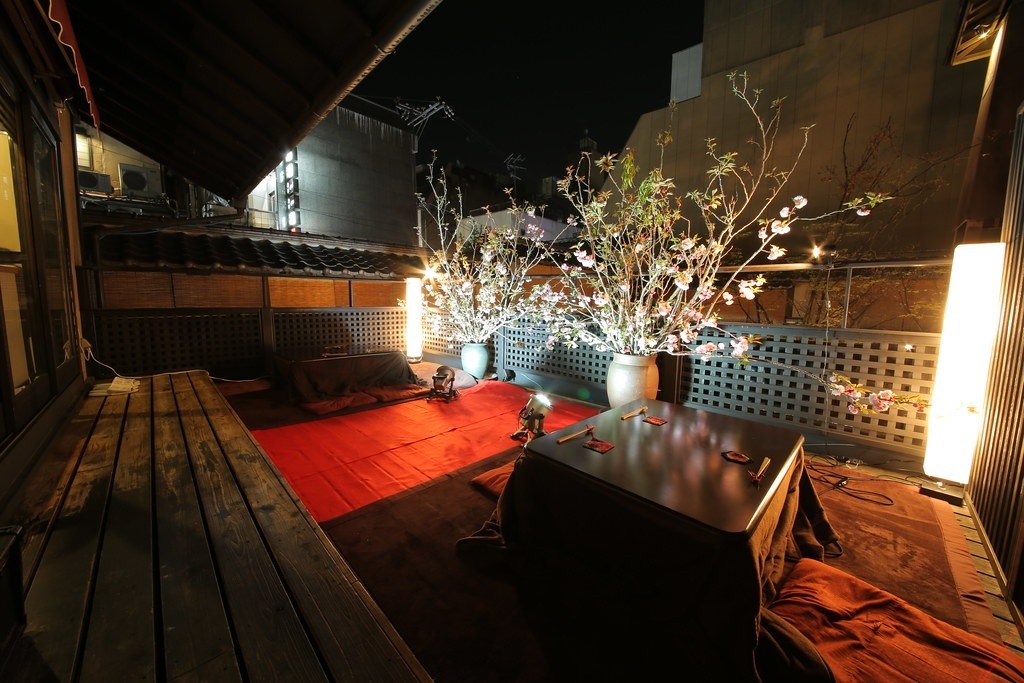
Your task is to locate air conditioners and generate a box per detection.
[118,162,163,199]
[78,169,112,195]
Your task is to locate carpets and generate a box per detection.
[322,431,1002,682]
[226,362,477,430]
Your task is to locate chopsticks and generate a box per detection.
[621,407,646,420]
[757,456,770,477]
[557,425,594,444]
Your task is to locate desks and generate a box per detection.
[273,346,425,403]
[457,395,841,683]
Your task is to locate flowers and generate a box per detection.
[395,149,572,348]
[526,68,980,421]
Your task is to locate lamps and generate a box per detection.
[428,365,462,404]
[510,392,553,447]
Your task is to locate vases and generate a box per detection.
[461,343,490,380]
[606,350,660,411]
